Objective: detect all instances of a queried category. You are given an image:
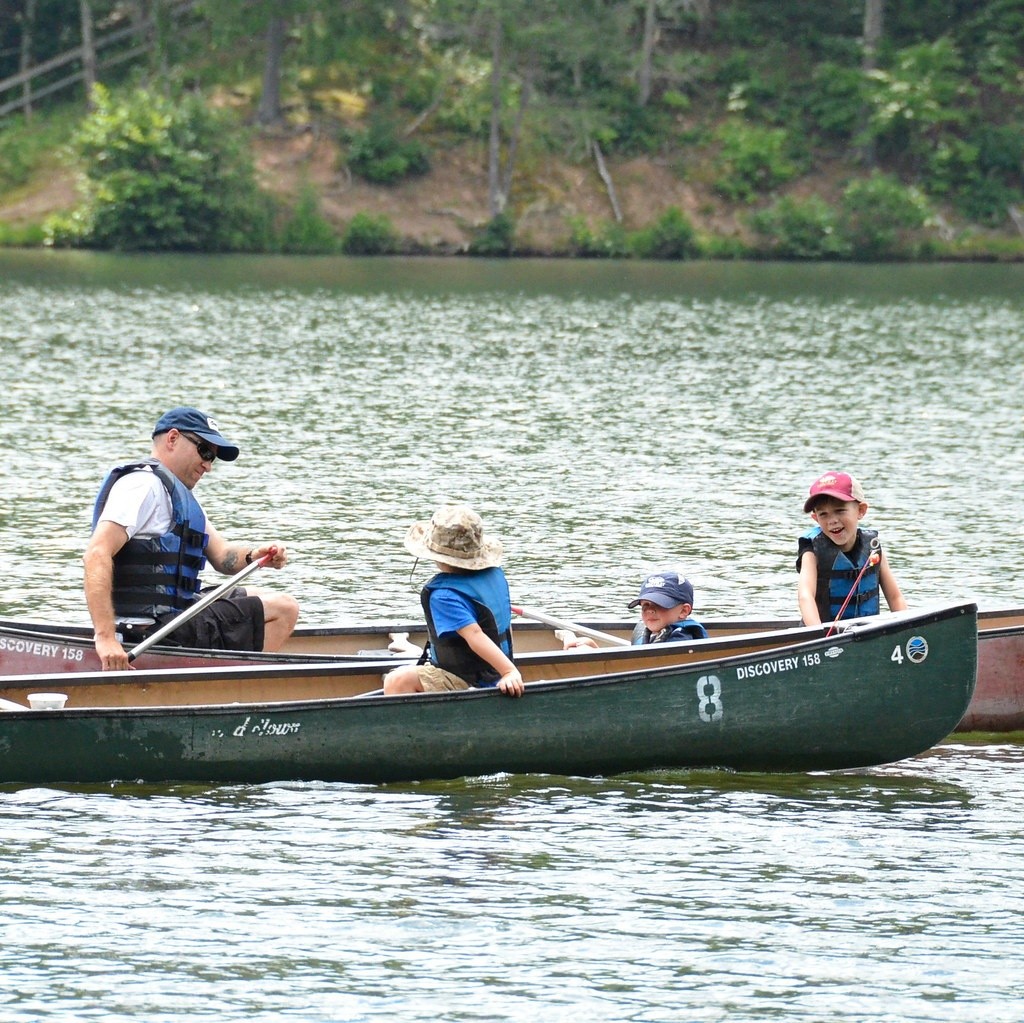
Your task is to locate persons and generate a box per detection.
[563,571,708,650]
[384,505,524,698]
[796,471,907,627]
[84,407,299,673]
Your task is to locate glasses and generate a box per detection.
[179,432,216,463]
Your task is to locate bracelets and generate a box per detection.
[246,549,253,565]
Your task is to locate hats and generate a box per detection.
[404,504,503,570]
[627,573,694,612]
[804,473,864,513]
[152,407,239,462]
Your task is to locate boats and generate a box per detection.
[0,603,979,787]
[0,608,1024,733]
